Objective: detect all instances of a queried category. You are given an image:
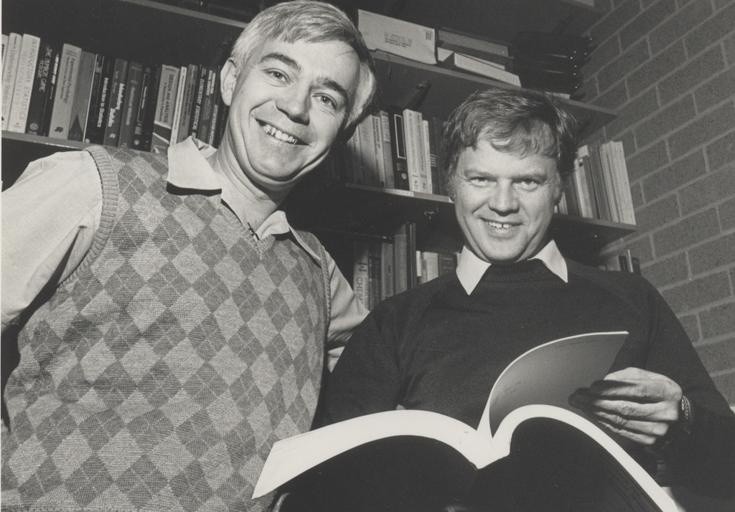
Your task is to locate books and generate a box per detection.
[436,25,522,87]
[338,223,456,311]
[252,330,682,508]
[318,109,449,196]
[2,32,225,154]
[555,140,634,225]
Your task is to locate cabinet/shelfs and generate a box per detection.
[0,1,646,310]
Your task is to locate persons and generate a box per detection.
[281,84,734,508]
[1,2,376,508]
